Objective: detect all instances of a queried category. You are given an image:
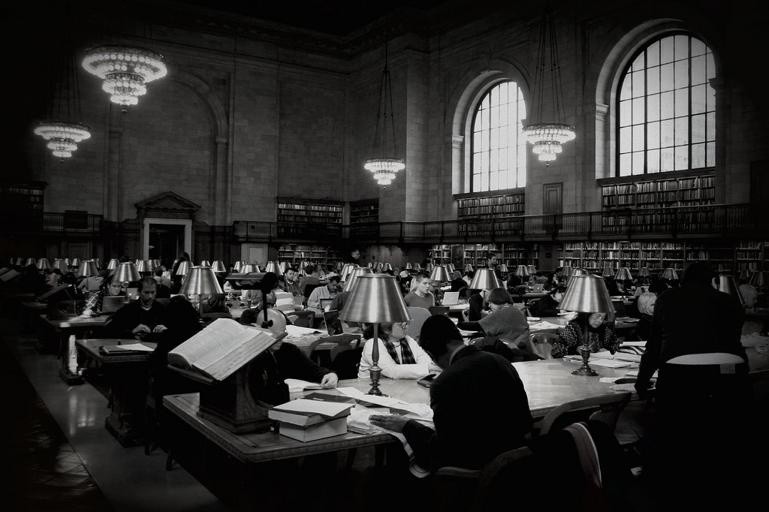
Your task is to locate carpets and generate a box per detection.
[7,332,766,511]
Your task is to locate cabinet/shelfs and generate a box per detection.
[597,168,715,238]
[431,246,534,269]
[560,242,768,286]
[278,196,379,241]
[279,244,340,264]
[453,188,527,238]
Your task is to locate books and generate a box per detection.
[456,196,524,240]
[278,201,379,240]
[601,174,715,235]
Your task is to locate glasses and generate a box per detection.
[395,322,408,328]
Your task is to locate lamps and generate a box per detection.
[521,1,576,168]
[30,0,170,162]
[360,1,409,189]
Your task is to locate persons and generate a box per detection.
[1,241,769,511]
